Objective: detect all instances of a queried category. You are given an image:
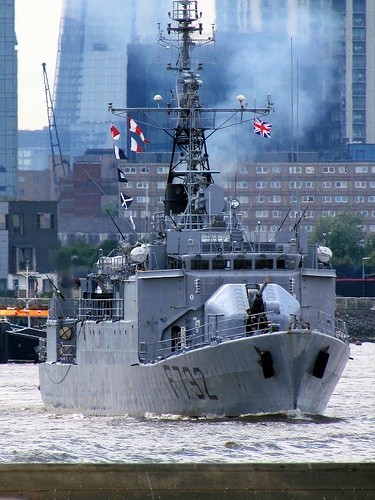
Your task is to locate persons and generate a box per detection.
[244,309,255,337]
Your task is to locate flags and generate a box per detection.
[120,193,133,210]
[117,168,128,182]
[110,115,151,159]
[253,117,272,139]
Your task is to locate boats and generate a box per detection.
[34,1,354,422]
